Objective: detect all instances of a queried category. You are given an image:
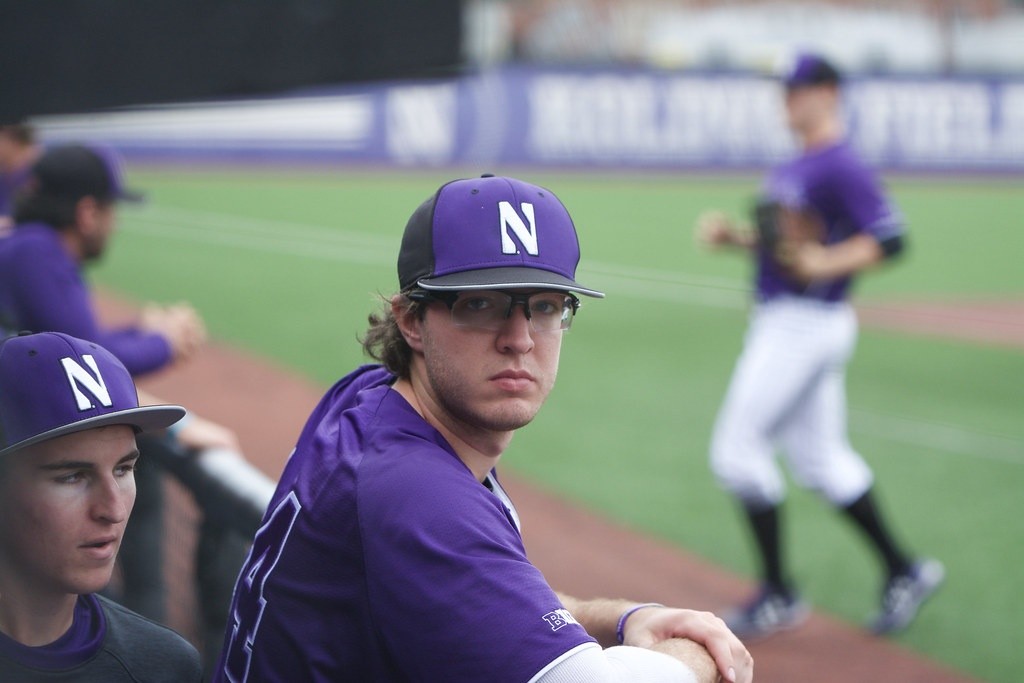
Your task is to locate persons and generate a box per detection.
[0,110,240,455]
[699,53,944,639]
[217,172,754,683]
[0,331,202,683]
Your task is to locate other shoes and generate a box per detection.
[871,559,943,636]
[724,590,805,640]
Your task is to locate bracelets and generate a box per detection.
[617,603,662,643]
[165,409,189,441]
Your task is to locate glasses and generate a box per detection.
[407,289,580,331]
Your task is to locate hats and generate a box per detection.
[781,56,843,88]
[397,173,605,300]
[0,330,187,458]
[32,143,145,203]
